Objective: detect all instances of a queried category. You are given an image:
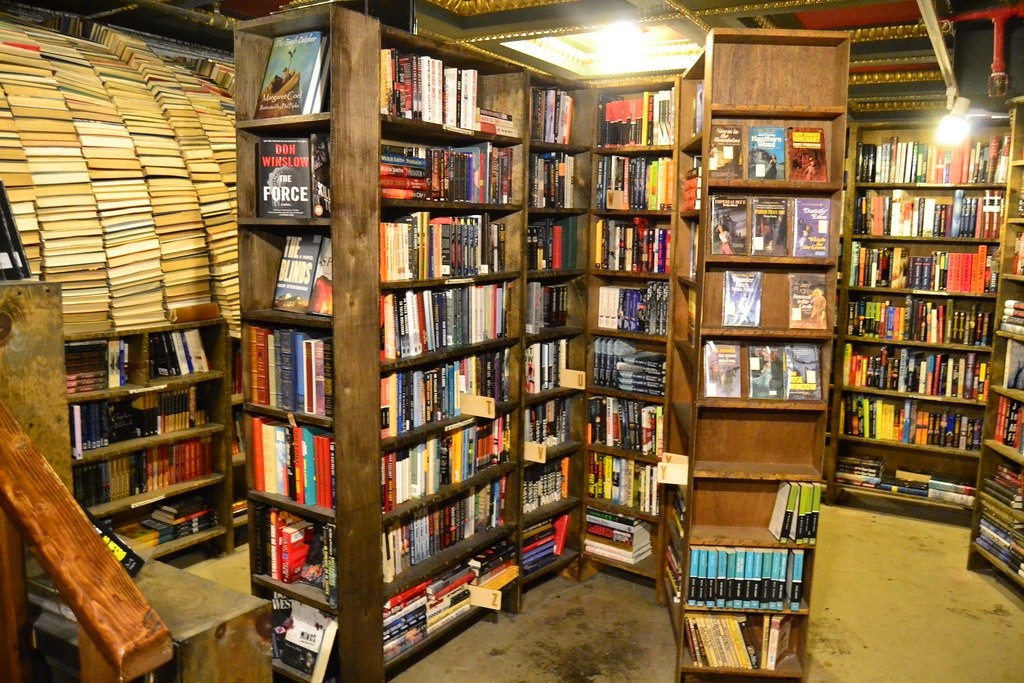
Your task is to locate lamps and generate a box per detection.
[935,87,971,144]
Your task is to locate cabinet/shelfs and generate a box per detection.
[0,0,1024,683]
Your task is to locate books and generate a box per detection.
[250,31,575,683]
[0,4,246,620]
[837,133,1024,576]
[583,85,831,670]
